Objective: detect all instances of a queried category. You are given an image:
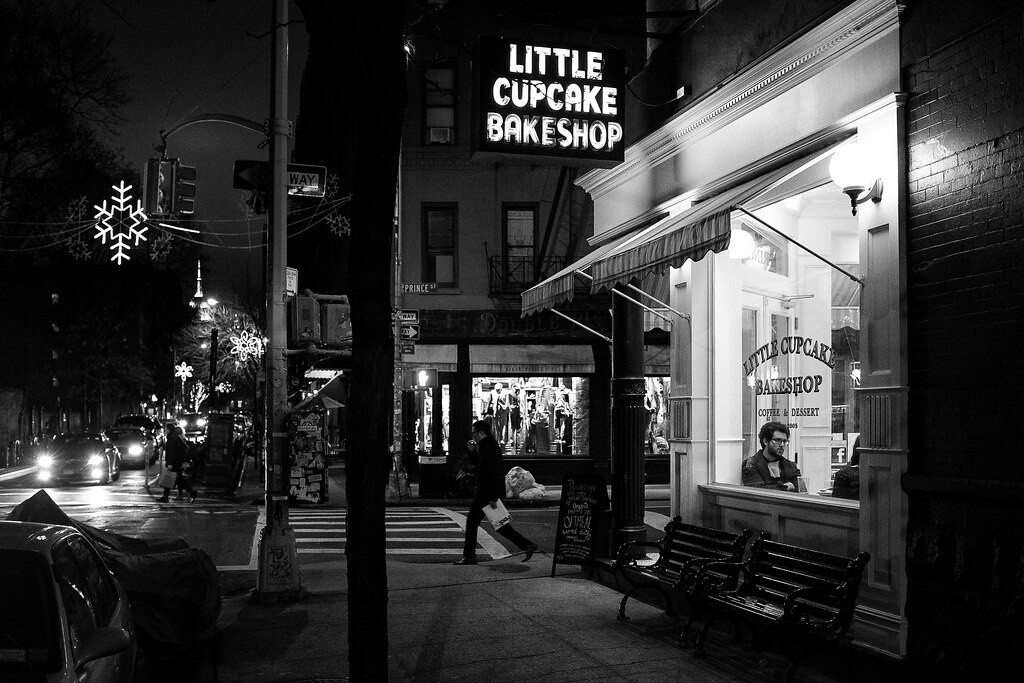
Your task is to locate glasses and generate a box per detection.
[772,437,791,444]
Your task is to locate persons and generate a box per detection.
[742,421,808,493]
[831,435,859,501]
[453,421,538,565]
[156,424,198,503]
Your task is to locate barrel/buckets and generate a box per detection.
[408,484,419,497]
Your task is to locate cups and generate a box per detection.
[824,480,834,490]
[797,476,810,494]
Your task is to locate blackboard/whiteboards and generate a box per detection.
[553,466,606,566]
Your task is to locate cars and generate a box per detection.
[105,426,157,468]
[0,519,138,682]
[178,413,209,436]
[39,431,122,484]
[113,415,167,457]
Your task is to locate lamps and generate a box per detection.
[828,141,884,218]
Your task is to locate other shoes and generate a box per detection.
[172,495,183,500]
[156,496,169,503]
[189,490,198,503]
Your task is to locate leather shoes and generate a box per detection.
[522,544,539,561]
[454,556,479,565]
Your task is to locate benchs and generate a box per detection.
[610,516,871,683]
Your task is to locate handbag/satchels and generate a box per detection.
[159,467,178,489]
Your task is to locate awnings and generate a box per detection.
[305,356,351,379]
[594,131,861,321]
[520,214,669,340]
